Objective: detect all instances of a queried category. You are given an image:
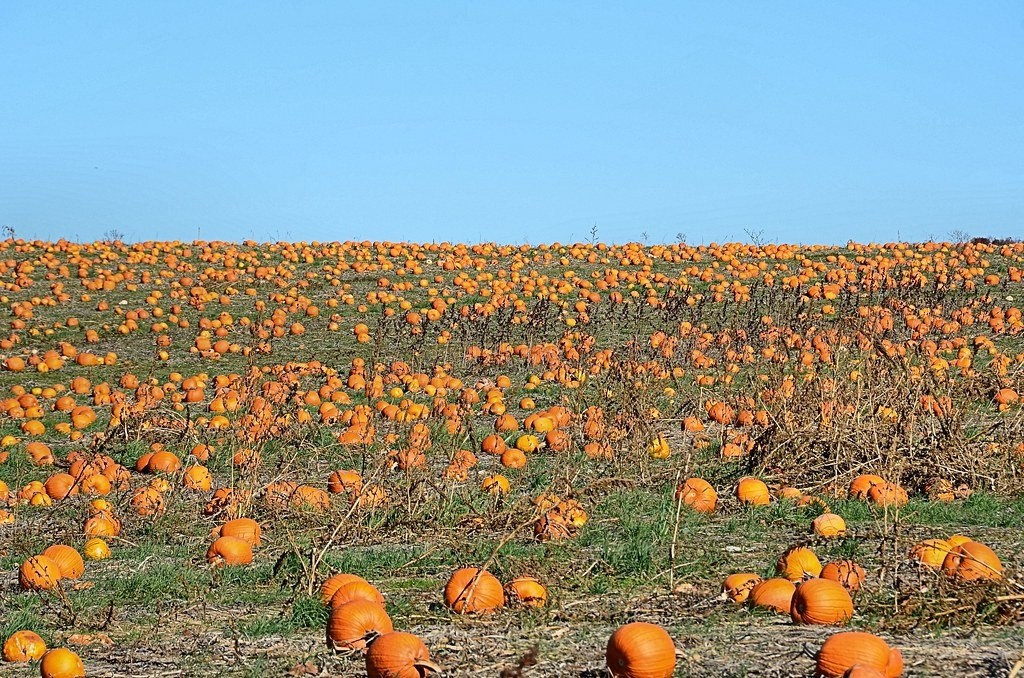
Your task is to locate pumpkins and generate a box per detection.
[0,240,1024,678]
